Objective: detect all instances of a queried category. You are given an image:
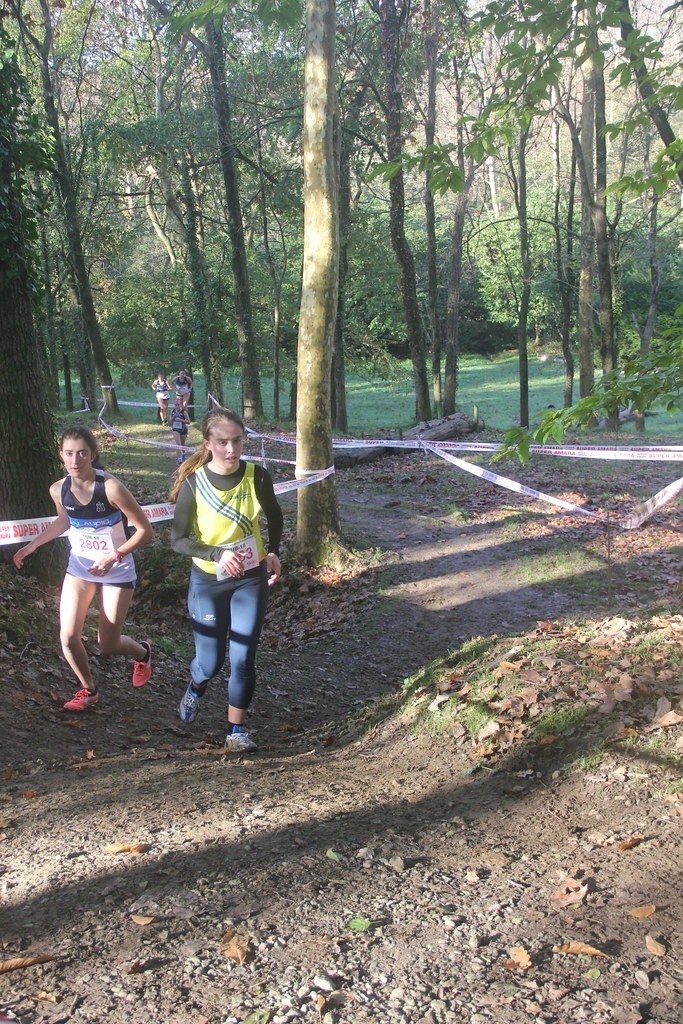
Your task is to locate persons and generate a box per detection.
[14,426,152,711]
[171,408,284,752]
[151,372,173,428]
[170,398,190,466]
[170,367,193,425]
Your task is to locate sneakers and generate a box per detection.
[63,687,99,711]
[182,455,185,462]
[226,733,258,752]
[178,458,182,464]
[161,420,169,426]
[179,679,204,722]
[132,641,151,687]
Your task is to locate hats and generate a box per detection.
[174,399,181,404]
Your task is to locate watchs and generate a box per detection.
[114,549,122,564]
